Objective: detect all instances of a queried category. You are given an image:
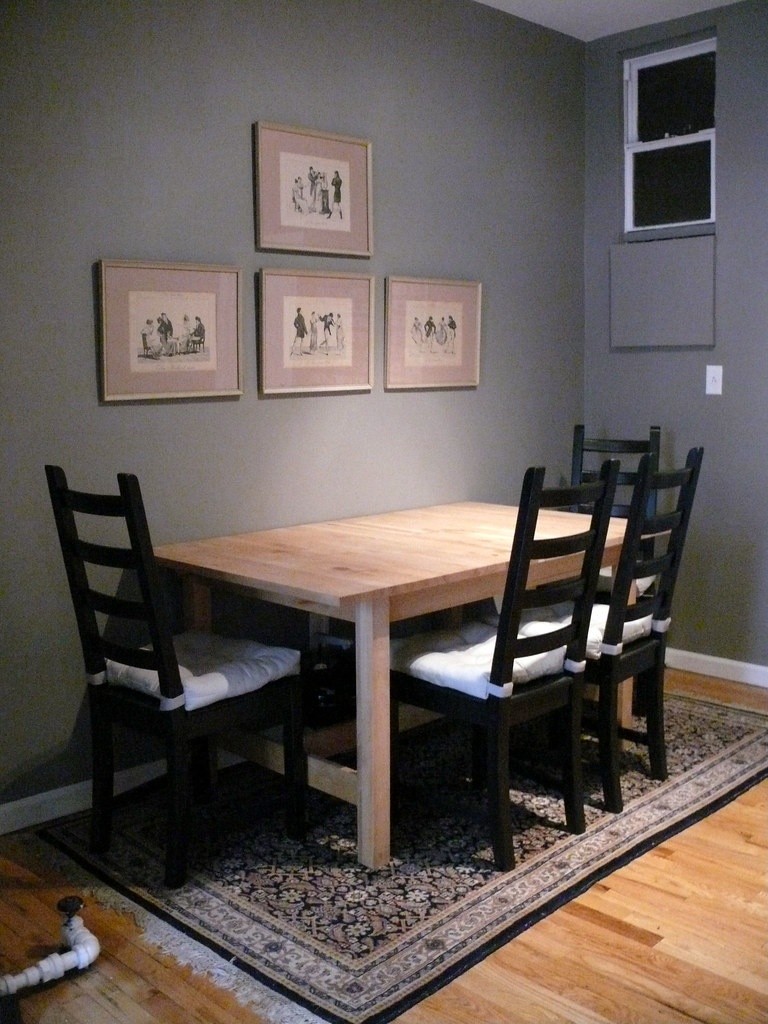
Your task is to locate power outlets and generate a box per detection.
[705,365,723,396]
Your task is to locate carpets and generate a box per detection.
[37,683,768,1024]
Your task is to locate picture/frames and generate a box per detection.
[384,275,483,392]
[96,258,245,405]
[253,118,374,258]
[257,267,375,396]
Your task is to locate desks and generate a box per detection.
[154,500,636,870]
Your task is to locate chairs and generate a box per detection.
[534,424,661,718]
[390,458,621,872]
[477,446,706,816]
[45,463,309,889]
